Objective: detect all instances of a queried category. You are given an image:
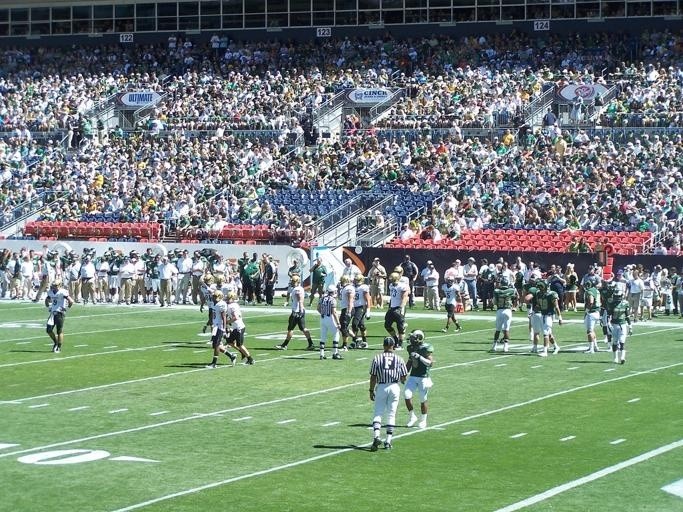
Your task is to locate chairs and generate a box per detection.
[374,130,501,144]
[249,188,364,218]
[230,130,282,142]
[383,237,455,249]
[0,212,163,239]
[182,219,283,246]
[454,222,656,254]
[365,181,443,218]
[579,127,682,143]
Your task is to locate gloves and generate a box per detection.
[628,326,633,337]
[410,352,421,361]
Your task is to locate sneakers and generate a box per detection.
[349,339,368,349]
[492,345,496,351]
[503,347,508,351]
[275,344,288,351]
[394,339,404,351]
[304,346,314,351]
[552,347,560,355]
[332,353,344,359]
[441,328,448,333]
[503,347,508,352]
[489,347,497,352]
[320,355,327,360]
[230,352,237,367]
[586,346,598,351]
[537,352,547,357]
[205,364,217,369]
[406,416,418,427]
[614,358,619,363]
[454,327,462,332]
[620,357,625,364]
[613,358,618,364]
[417,420,427,429]
[528,348,537,353]
[53,343,62,353]
[340,346,348,351]
[244,359,255,366]
[239,355,248,365]
[584,349,595,354]
[371,437,382,452]
[384,442,393,449]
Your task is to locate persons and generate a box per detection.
[149,253,161,303]
[524,279,545,354]
[618,268,630,301]
[192,251,208,304]
[107,255,122,301]
[441,276,462,333]
[628,270,640,322]
[482,264,495,312]
[368,257,387,309]
[78,256,97,306]
[19,254,36,302]
[477,258,490,303]
[94,256,111,303]
[603,284,633,364]
[563,265,579,314]
[317,284,344,360]
[224,291,255,366]
[339,274,363,350]
[0,129,683,250]
[547,263,562,280]
[513,264,524,312]
[156,257,179,307]
[275,276,315,351]
[64,255,82,305]
[347,273,370,350]
[498,257,505,263]
[202,290,238,369]
[132,253,147,304]
[398,254,418,308]
[405,331,435,430]
[50,250,64,288]
[548,270,565,313]
[652,264,683,319]
[243,252,263,306]
[5,252,24,298]
[581,262,616,285]
[340,259,363,287]
[237,250,250,285]
[261,252,276,307]
[557,265,564,278]
[527,280,564,359]
[640,270,654,320]
[512,256,528,273]
[369,337,408,453]
[1,27,683,130]
[496,264,503,275]
[394,267,411,332]
[384,273,410,352]
[30,255,60,303]
[141,248,157,303]
[600,281,615,353]
[117,256,136,306]
[198,257,243,290]
[308,259,327,308]
[497,262,517,311]
[0,247,10,296]
[171,249,192,304]
[487,279,516,353]
[463,257,480,312]
[454,259,464,281]
[424,263,441,312]
[452,276,469,312]
[44,283,72,353]
[444,262,462,315]
[524,261,534,313]
[284,260,304,307]
[420,260,435,310]
[582,278,602,355]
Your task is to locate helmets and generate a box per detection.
[389,272,401,284]
[292,275,301,284]
[411,329,425,344]
[214,274,226,284]
[500,279,509,289]
[536,280,548,291]
[204,275,214,285]
[582,281,592,290]
[213,290,223,302]
[339,276,350,286]
[393,266,404,277]
[354,274,364,284]
[226,292,236,301]
[53,280,61,289]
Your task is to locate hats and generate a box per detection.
[328,285,338,292]
[383,337,395,347]
